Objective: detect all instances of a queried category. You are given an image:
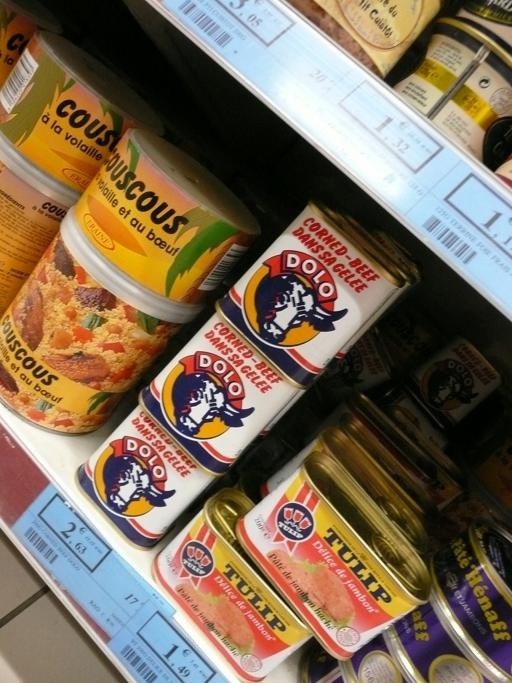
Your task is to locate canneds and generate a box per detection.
[289,0,441,82]
[388,16,512,167]
[0,0,333,683]
[335,207,512,682]
